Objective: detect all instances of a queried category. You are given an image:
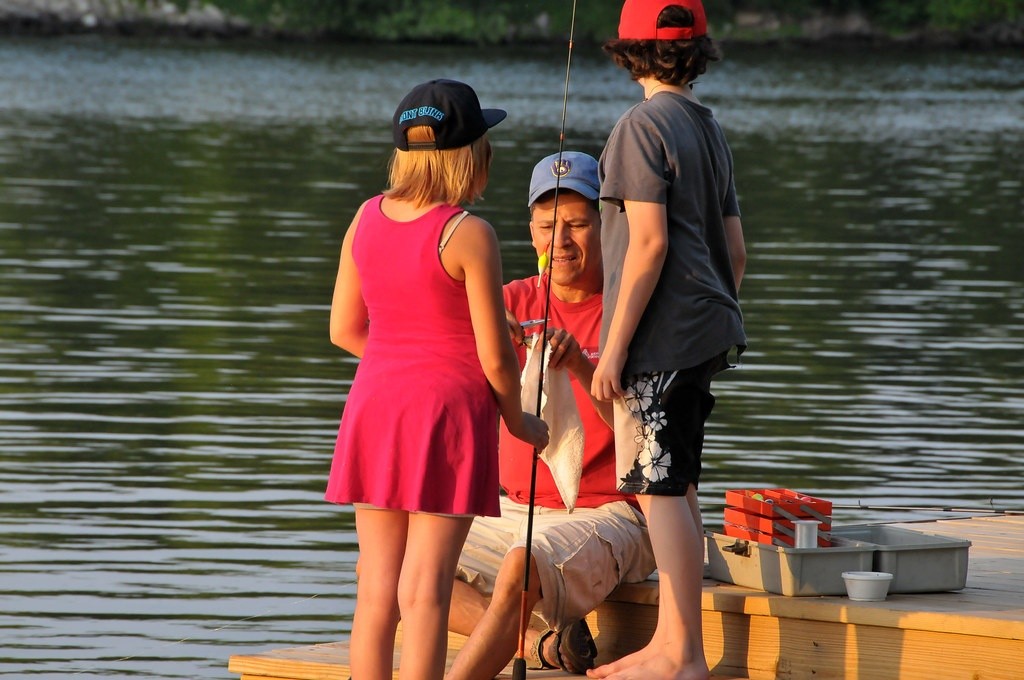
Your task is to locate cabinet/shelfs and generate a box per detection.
[724,487,834,547]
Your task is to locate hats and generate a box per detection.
[618,0,707,40]
[393,80,507,151]
[528,151,600,207]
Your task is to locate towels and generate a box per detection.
[517,331,586,516]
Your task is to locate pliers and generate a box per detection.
[521,317,552,329]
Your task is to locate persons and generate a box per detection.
[321,77,547,680]
[589,0,750,679]
[455,150,655,671]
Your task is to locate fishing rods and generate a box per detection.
[509,0,580,679]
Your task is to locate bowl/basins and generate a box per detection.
[841,571,893,601]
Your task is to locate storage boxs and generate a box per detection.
[704,530,877,601]
[834,525,972,595]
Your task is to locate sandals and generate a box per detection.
[527,618,598,675]
